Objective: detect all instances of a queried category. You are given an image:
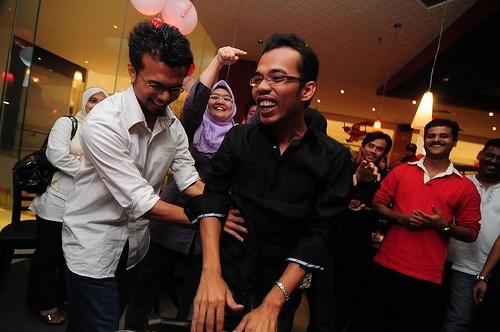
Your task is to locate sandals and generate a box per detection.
[38,306,66,326]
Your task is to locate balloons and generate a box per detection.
[161,0,198,36]
[129,0,168,16]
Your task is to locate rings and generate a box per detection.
[224,49,229,53]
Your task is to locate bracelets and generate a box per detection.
[274,280,291,302]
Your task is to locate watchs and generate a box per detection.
[474,274,489,283]
[441,223,452,233]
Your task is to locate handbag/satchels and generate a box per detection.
[12,114,78,197]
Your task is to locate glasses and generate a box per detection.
[249,73,303,87]
[209,94,234,102]
[139,71,185,93]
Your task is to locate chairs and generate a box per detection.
[0,170,43,287]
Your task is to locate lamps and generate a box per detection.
[412,0,450,129]
[370,22,402,133]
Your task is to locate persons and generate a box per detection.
[23,87,111,324]
[122,44,500,332]
[184,32,353,332]
[62,19,248,332]
